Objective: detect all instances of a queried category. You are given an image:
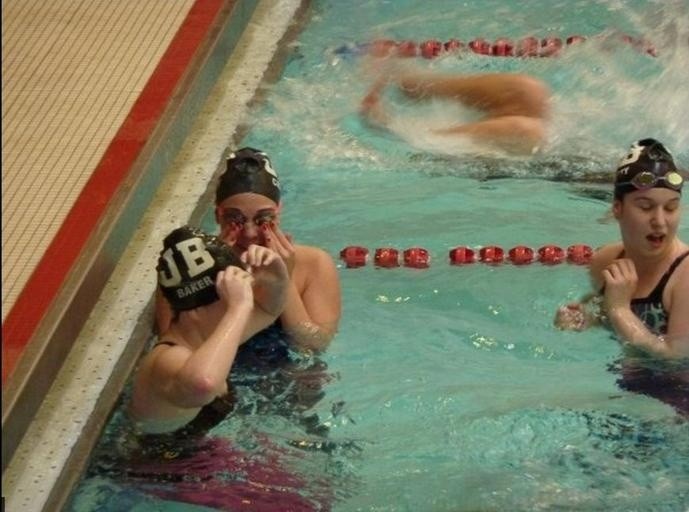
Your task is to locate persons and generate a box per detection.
[132,224,291,451]
[154,146,341,358]
[551,138,688,366]
[353,47,557,149]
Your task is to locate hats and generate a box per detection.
[156,226,247,314]
[214,147,283,206]
[614,138,684,199]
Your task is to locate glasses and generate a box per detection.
[618,170,684,191]
[220,208,278,228]
